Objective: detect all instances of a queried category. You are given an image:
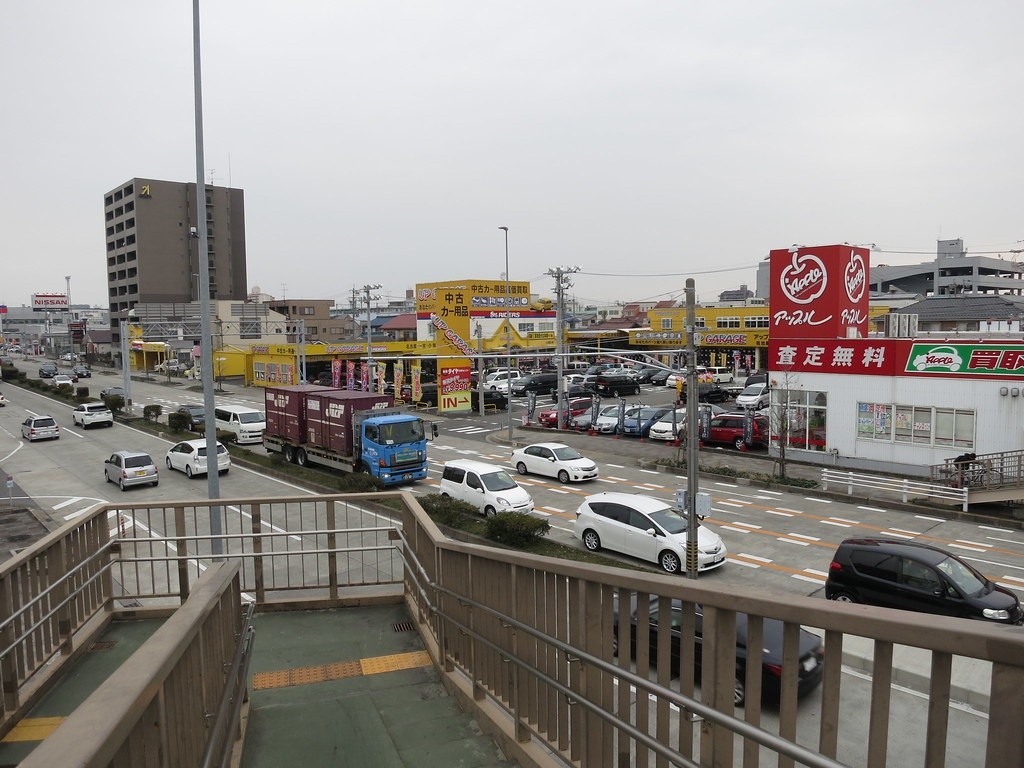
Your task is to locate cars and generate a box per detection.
[385,382,395,391]
[568,405,621,431]
[497,377,523,394]
[20,415,59,442]
[574,492,728,573]
[649,407,716,442]
[4,359,13,366]
[39,365,59,378]
[471,390,508,411]
[471,374,479,389]
[510,442,599,484]
[8,345,25,353]
[650,370,671,385]
[65,353,77,361]
[563,375,582,390]
[166,438,231,479]
[105,449,159,491]
[56,370,78,383]
[175,405,205,431]
[354,379,378,391]
[618,370,641,380]
[592,406,650,434]
[601,368,625,375]
[409,385,438,407]
[551,385,596,401]
[100,387,125,404]
[51,375,73,386]
[315,372,347,388]
[735,383,769,410]
[618,409,676,438]
[72,366,91,378]
[631,368,660,384]
[401,387,411,401]
[0,392,6,406]
[184,366,200,377]
[600,594,825,708]
[666,368,707,388]
[678,403,729,416]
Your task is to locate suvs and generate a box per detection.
[680,382,729,404]
[745,374,768,390]
[698,412,770,451]
[538,397,592,430]
[73,403,113,430]
[153,360,179,372]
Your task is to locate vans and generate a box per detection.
[214,405,266,444]
[600,364,613,369]
[586,366,608,376]
[511,373,557,396]
[595,376,640,398]
[477,371,521,391]
[614,363,634,372]
[572,376,597,390]
[707,367,733,384]
[488,367,524,377]
[439,458,535,519]
[825,536,1024,625]
[567,362,590,372]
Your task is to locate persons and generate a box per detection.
[952,453,977,486]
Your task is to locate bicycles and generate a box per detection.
[949,461,1003,493]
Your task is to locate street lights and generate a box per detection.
[498,226,513,440]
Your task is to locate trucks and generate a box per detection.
[262,385,439,487]
